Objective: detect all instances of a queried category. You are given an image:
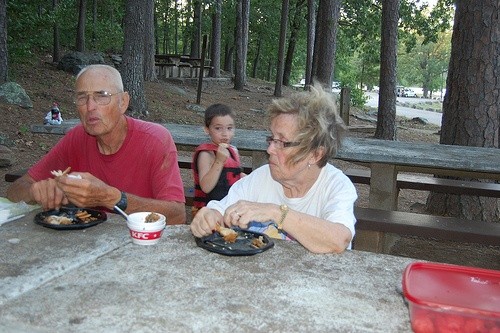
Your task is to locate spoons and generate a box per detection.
[204,240,240,252]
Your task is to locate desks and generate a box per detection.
[29,118,500,225]
[1,228,426,333]
[0,197,131,305]
[155,53,211,77]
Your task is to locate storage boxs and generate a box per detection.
[401,260,500,333]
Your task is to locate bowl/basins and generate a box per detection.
[127,212,167,245]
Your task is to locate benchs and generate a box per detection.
[177,157,500,254]
[156,63,215,69]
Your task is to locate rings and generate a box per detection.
[235,211,241,217]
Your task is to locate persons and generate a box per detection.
[7,64,186,225]
[192,103,247,209]
[190,83,358,253]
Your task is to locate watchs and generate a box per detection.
[280,205,288,224]
[113,192,127,214]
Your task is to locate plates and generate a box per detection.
[35,208,107,231]
[195,227,275,257]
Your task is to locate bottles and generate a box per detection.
[51,103,59,122]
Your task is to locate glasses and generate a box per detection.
[73,92,124,106]
[265,135,301,149]
